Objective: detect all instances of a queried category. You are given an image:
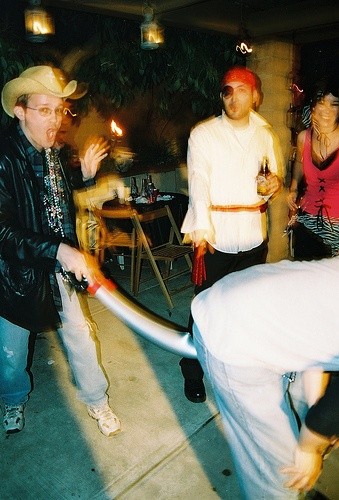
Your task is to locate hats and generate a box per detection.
[2,65,76,118]
[221,68,260,90]
[52,68,87,100]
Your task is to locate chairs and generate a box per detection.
[93,209,152,290]
[127,202,194,312]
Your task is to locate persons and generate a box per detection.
[179,68,285,403]
[0,66,121,435]
[191,255,339,500]
[287,86,339,262]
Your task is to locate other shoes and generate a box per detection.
[305,489,330,500]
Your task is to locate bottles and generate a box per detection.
[139,175,154,199]
[257,156,272,195]
[131,176,138,199]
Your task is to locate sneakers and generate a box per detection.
[88,400,120,437]
[3,404,24,433]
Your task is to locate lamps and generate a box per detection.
[139,4,164,51]
[23,0,56,42]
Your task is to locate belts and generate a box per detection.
[212,199,266,212]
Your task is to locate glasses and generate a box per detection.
[25,105,65,118]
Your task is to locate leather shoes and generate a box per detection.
[180,364,206,403]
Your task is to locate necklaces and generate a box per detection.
[313,122,338,159]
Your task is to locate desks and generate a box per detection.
[97,192,187,272]
